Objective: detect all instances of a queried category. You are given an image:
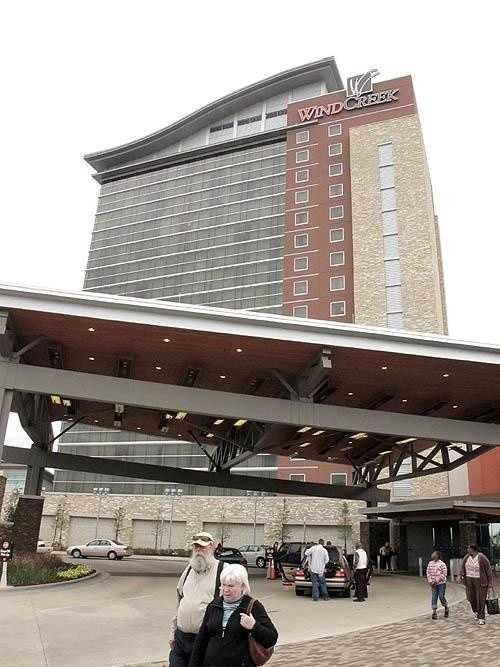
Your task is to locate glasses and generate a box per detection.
[193,536,213,542]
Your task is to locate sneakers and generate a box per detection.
[432,608,485,625]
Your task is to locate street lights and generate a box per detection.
[92,485,110,539]
[247,489,266,544]
[164,486,183,554]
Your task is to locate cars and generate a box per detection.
[66,537,133,560]
[36,536,55,553]
[277,539,374,598]
[213,547,249,573]
[235,544,274,566]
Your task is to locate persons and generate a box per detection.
[188,563,278,666]
[302,541,315,571]
[305,539,330,601]
[352,542,369,602]
[378,541,392,570]
[169,532,229,667]
[327,541,331,545]
[426,551,450,619]
[457,544,492,625]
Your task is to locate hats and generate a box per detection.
[192,532,215,547]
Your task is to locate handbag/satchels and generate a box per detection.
[249,633,274,666]
[486,599,500,614]
